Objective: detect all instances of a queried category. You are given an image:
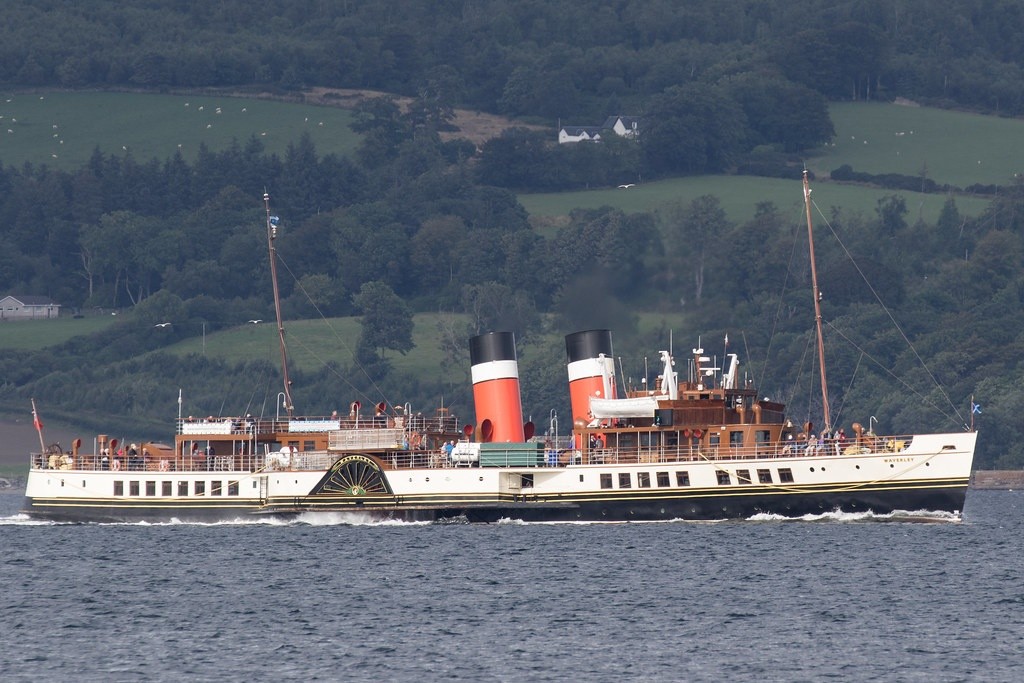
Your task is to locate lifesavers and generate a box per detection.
[110,460,121,471]
[411,433,422,448]
[158,461,169,472]
[543,440,553,452]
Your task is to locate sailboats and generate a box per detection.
[20,165,977,525]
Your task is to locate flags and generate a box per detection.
[33,414,44,430]
[270,216,279,225]
[973,399,982,413]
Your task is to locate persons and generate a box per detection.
[817,435,824,451]
[804,435,817,456]
[441,440,455,454]
[142,447,151,463]
[98,448,109,469]
[246,413,256,422]
[128,443,138,470]
[209,415,215,422]
[824,428,846,454]
[187,416,195,423]
[783,435,793,453]
